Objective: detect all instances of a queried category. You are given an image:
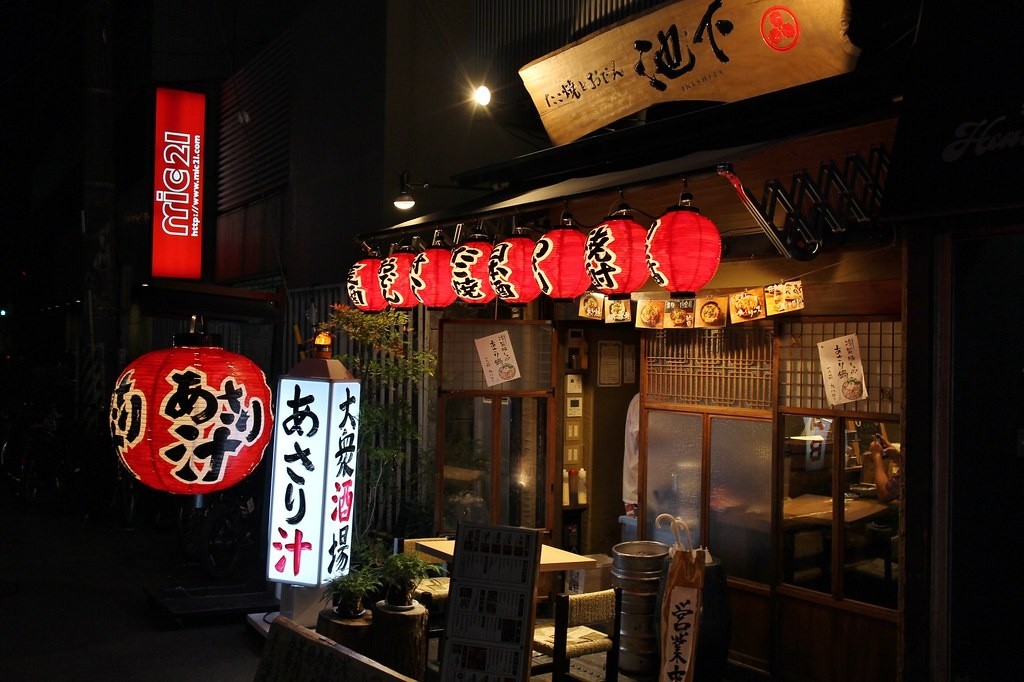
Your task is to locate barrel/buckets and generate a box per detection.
[608,540,670,672]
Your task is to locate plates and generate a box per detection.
[700,301,721,323]
[640,306,659,326]
[669,308,687,325]
[582,295,600,317]
[609,304,628,321]
[842,378,863,400]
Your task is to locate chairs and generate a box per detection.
[531,586,622,682]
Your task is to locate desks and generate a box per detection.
[416,535,598,594]
[781,491,889,592]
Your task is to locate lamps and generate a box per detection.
[392,172,495,211]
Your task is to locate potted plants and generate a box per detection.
[376,552,450,610]
[319,560,384,620]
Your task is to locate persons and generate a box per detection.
[622,390,675,516]
[870,433,899,503]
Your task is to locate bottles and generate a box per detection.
[562,469,570,506]
[577,467,587,505]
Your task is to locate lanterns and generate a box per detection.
[346,206,720,315]
[108,333,276,510]
